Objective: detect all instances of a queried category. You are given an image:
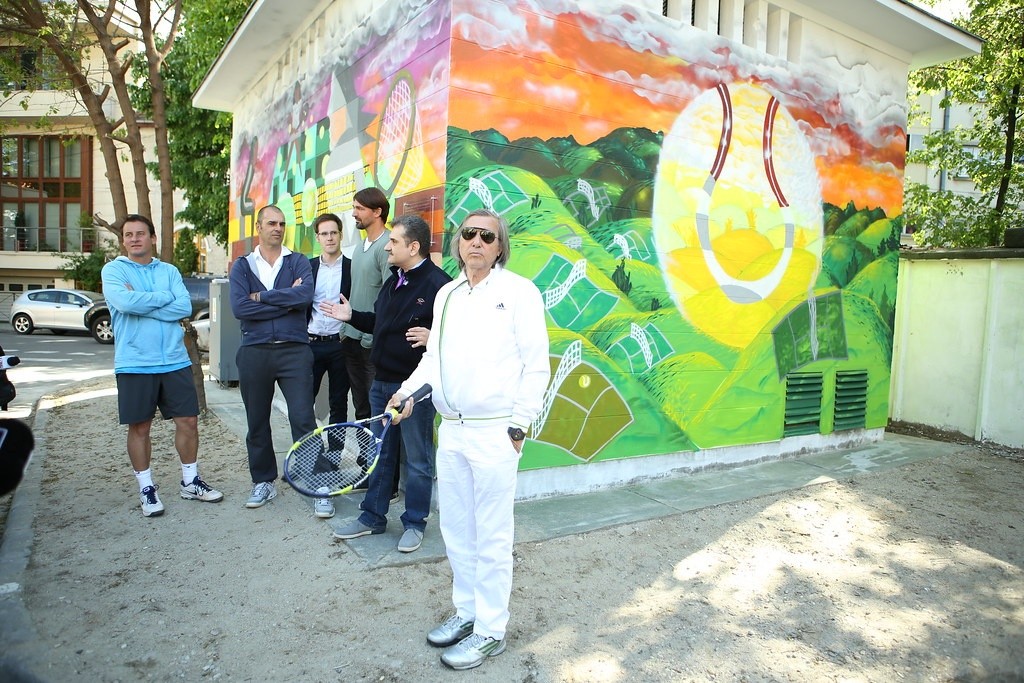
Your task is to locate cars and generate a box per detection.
[9,287,104,336]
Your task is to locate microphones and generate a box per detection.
[0,354,20,370]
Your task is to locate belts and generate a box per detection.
[309,334,340,342]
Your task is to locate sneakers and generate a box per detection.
[441,633,506,670]
[139,484,165,516]
[427,614,474,646]
[313,497,334,518]
[180,476,223,502]
[334,520,385,539]
[398,529,424,552]
[246,480,277,508]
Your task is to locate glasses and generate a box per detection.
[318,231,342,237]
[461,227,499,244]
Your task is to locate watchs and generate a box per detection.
[508,427,526,440]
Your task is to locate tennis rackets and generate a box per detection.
[283,382,433,500]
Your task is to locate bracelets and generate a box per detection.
[255,294,257,300]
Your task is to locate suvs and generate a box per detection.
[83,270,230,345]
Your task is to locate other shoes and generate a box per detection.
[390,493,399,502]
[351,480,368,493]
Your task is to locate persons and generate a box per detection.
[338,187,399,509]
[383,211,550,669]
[229,206,337,516]
[281,212,351,482]
[100,216,223,516]
[318,216,454,551]
